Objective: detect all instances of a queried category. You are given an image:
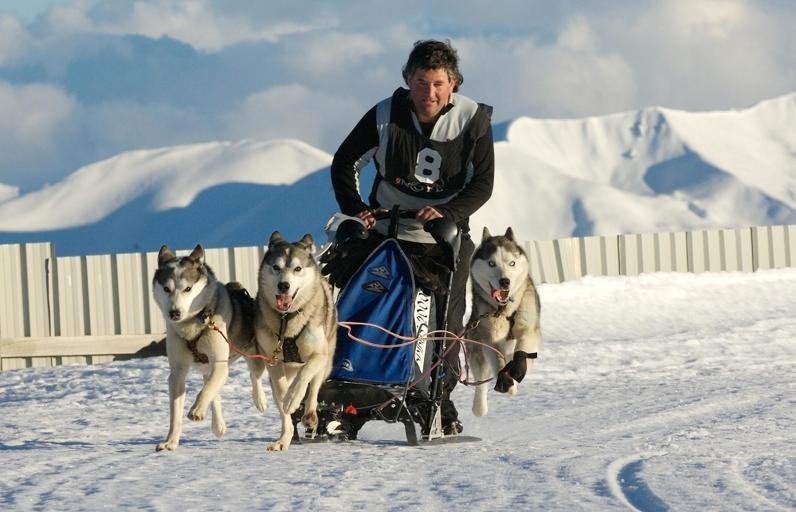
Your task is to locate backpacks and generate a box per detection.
[323,235,438,392]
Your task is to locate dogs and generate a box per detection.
[253,230,338,451]
[152,244,267,452]
[464,226,542,418]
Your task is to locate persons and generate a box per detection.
[300,37,497,444]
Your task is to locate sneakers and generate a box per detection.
[420,400,463,438]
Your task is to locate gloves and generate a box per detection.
[397,237,452,298]
[318,226,382,288]
